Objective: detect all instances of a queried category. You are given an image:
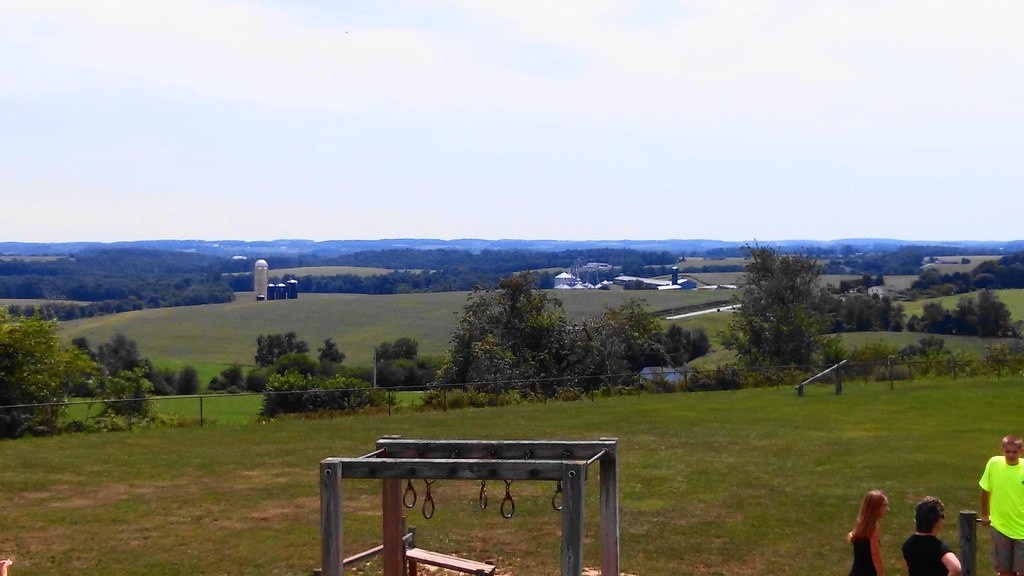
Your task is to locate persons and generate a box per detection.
[846,490,890,576]
[901,497,962,576]
[978,435,1023,576]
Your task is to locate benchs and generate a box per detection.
[406,546,496,576]
